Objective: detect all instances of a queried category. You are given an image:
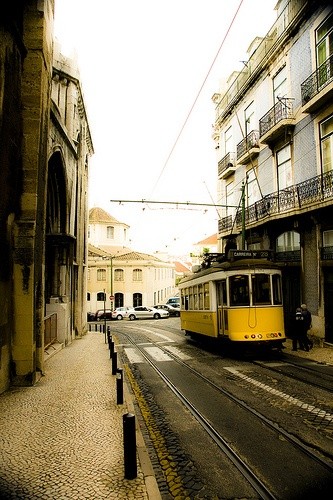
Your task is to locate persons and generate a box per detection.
[301,304,313,352]
[291,307,305,352]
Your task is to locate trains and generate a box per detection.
[177,256,290,354]
[289,305,291,306]
[168,295,181,308]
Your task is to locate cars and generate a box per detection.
[125,305,170,321]
[112,305,134,320]
[86,312,96,322]
[154,304,181,318]
[95,309,115,321]
